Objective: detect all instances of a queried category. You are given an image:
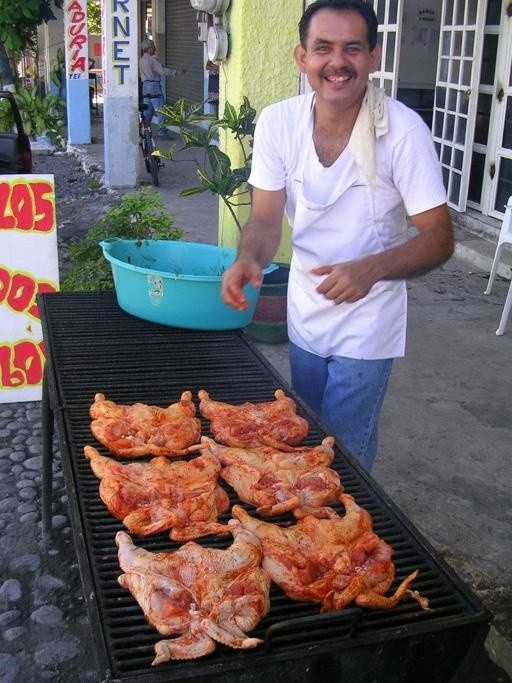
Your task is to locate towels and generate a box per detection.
[349,82,388,186]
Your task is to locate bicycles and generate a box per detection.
[139,92,164,186]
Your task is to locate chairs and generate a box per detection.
[483,196,512,336]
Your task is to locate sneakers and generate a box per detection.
[158,131,170,138]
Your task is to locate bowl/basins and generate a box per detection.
[97,236,280,331]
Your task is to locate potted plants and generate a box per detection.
[150,94,291,342]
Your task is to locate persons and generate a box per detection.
[220,0,455,476]
[138,39,187,140]
[88,58,98,110]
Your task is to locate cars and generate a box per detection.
[0,90,33,173]
